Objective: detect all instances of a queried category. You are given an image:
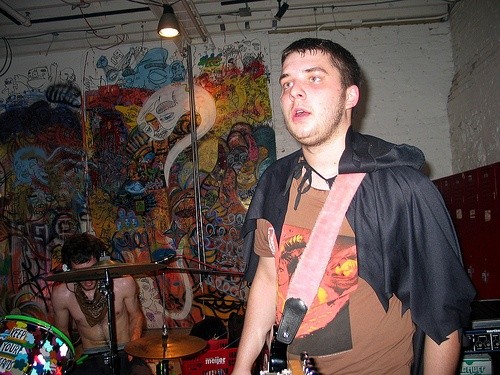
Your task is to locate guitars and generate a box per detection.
[260,324,318,375]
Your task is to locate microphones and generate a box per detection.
[155,255,175,264]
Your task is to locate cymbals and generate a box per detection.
[124,332,208,358]
[41,263,168,283]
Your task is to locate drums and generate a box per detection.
[0,315,75,375]
[80,344,130,375]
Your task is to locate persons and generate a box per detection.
[230,38,480,375]
[50,232,155,375]
